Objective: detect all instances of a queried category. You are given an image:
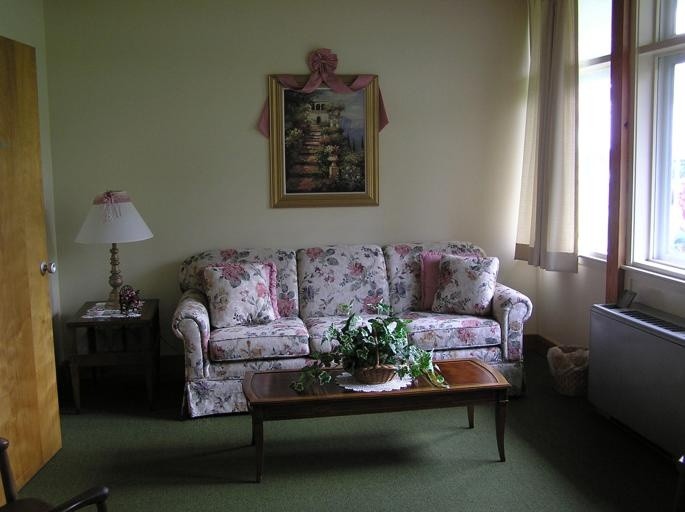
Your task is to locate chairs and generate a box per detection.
[0,438,108,512]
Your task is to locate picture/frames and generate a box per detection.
[268,72,379,209]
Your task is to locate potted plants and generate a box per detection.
[288,296,449,395]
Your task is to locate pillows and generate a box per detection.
[201,263,281,320]
[432,254,500,317]
[420,252,482,312]
[203,261,276,328]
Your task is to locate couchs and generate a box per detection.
[171,241,533,421]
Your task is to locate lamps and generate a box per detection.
[74,202,154,309]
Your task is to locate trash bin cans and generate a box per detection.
[548,344,589,397]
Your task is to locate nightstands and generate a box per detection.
[67,299,162,414]
